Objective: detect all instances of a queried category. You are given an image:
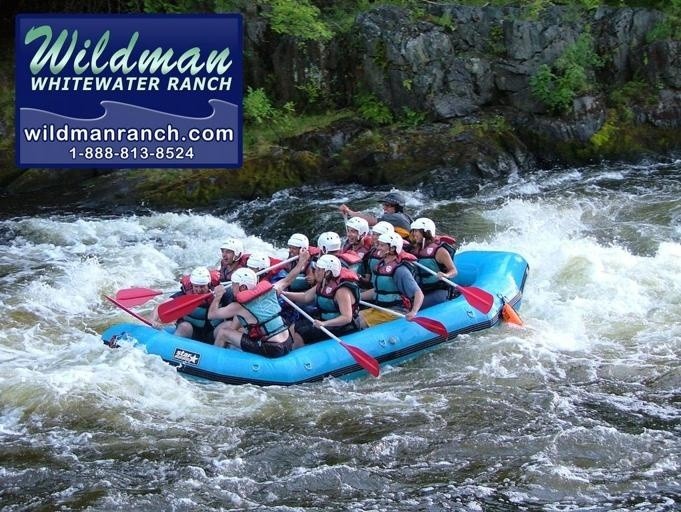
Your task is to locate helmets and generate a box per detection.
[230,267,258,290]
[317,231,341,256]
[220,238,244,262]
[287,233,310,251]
[376,193,405,207]
[247,251,271,273]
[346,217,403,255]
[190,267,212,286]
[316,254,342,278]
[410,217,435,238]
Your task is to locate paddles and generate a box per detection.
[415,261,494,313]
[118,290,180,309]
[360,300,449,337]
[102,293,168,330]
[157,255,298,323]
[280,294,380,378]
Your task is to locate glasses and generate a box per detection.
[383,202,389,207]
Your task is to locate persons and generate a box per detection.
[206,247,312,359]
[218,193,458,340]
[151,266,217,344]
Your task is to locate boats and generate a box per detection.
[101,250,531,387]
